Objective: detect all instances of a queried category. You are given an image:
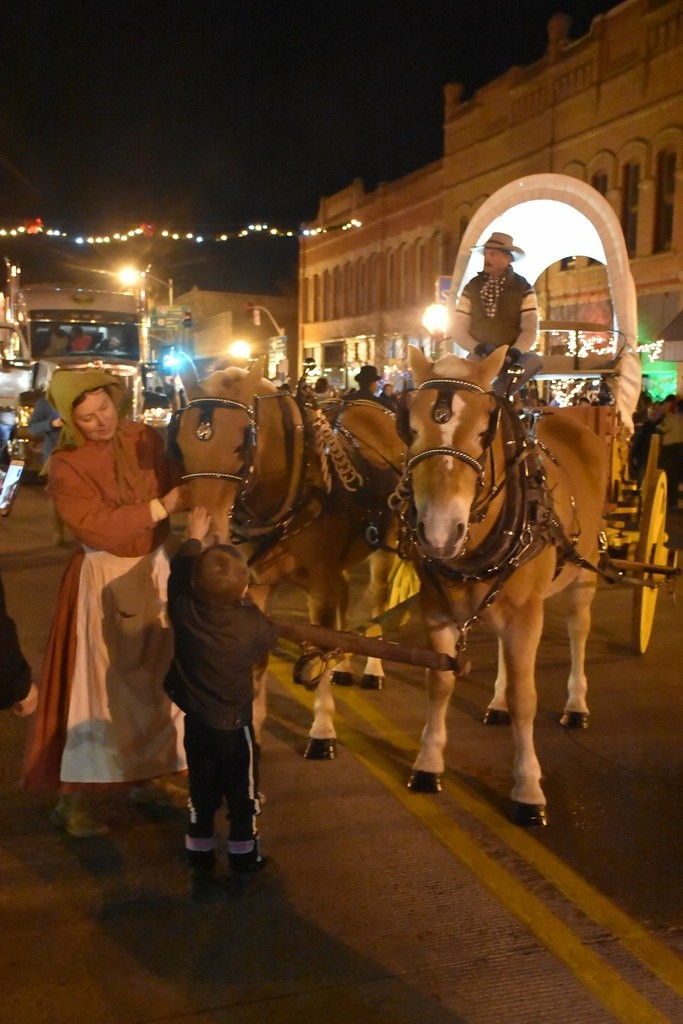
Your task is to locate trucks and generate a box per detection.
[0,281,181,485]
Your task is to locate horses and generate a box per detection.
[175,350,407,753]
[405,342,610,829]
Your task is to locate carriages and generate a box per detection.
[153,173,682,827]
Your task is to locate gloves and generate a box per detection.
[474,343,497,358]
[503,349,521,366]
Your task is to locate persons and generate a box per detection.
[0,579,36,717]
[161,506,271,874]
[141,361,683,512]
[450,231,537,357]
[16,380,189,838]
[25,389,64,545]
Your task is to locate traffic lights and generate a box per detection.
[157,345,182,378]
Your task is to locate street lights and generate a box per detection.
[245,302,286,337]
[421,303,449,362]
[117,265,175,307]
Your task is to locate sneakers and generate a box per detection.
[134,784,191,807]
[63,807,111,836]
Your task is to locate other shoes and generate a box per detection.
[231,831,271,870]
[184,835,213,874]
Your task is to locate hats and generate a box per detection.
[354,364,380,383]
[470,231,526,261]
